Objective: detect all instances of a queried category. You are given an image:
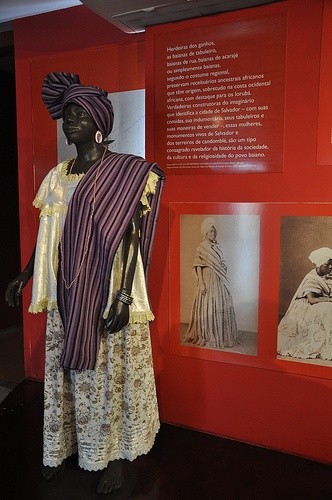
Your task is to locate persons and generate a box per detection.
[277,247,332,362]
[5,84,160,495]
[185,217,241,348]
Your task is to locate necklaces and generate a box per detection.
[60,144,107,289]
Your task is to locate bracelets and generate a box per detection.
[116,290,134,305]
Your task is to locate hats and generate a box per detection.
[201,217,217,236]
[309,247,332,268]
